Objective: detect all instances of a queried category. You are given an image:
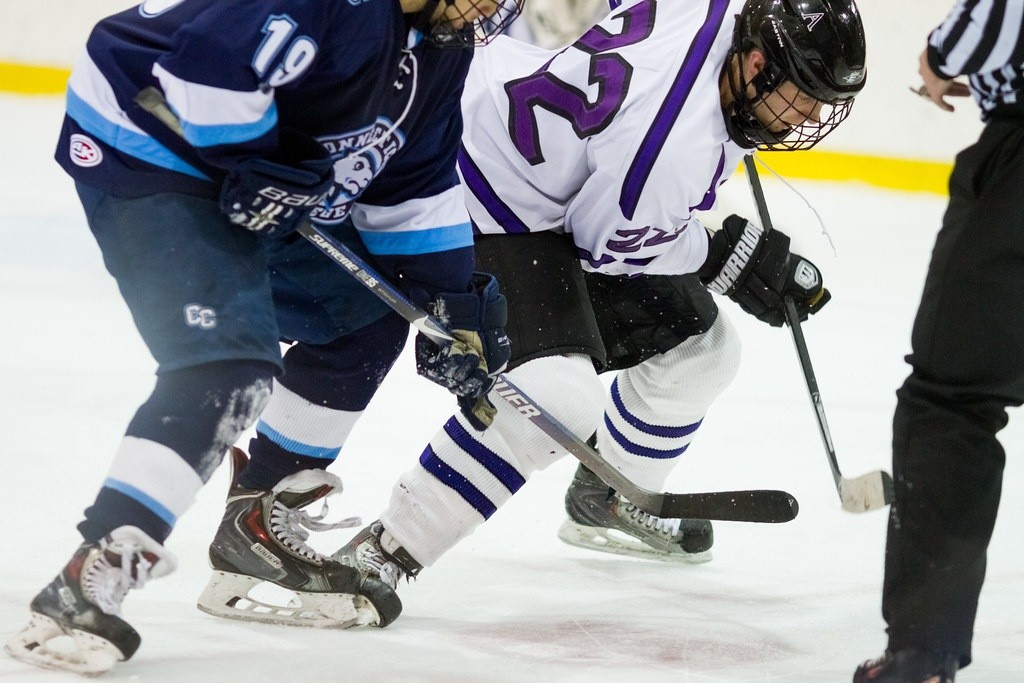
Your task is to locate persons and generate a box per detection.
[332,0,866,596]
[854,0,1024,683]
[3,0,524,675]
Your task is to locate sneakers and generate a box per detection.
[2,526,180,677]
[331,521,424,628]
[853,646,959,683]
[196,442,361,630]
[558,432,714,563]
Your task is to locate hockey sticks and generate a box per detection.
[741,149,898,516]
[133,83,804,524]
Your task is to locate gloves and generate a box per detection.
[697,214,831,328]
[219,132,335,241]
[415,273,513,432]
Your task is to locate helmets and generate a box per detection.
[733,0,868,104]
